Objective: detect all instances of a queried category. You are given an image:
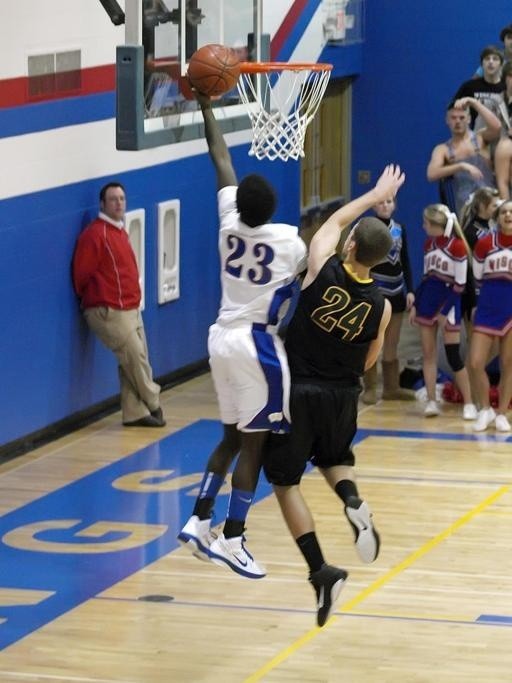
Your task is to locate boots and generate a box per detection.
[361,359,416,405]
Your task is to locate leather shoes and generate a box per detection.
[122,407,166,426]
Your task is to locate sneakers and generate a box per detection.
[308,563,347,626]
[344,495,379,564]
[177,515,268,579]
[423,401,512,432]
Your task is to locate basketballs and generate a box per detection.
[188,43,241,97]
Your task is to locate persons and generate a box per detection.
[360,193,416,405]
[265,162,409,630]
[72,179,168,427]
[177,84,307,582]
[411,25,512,431]
